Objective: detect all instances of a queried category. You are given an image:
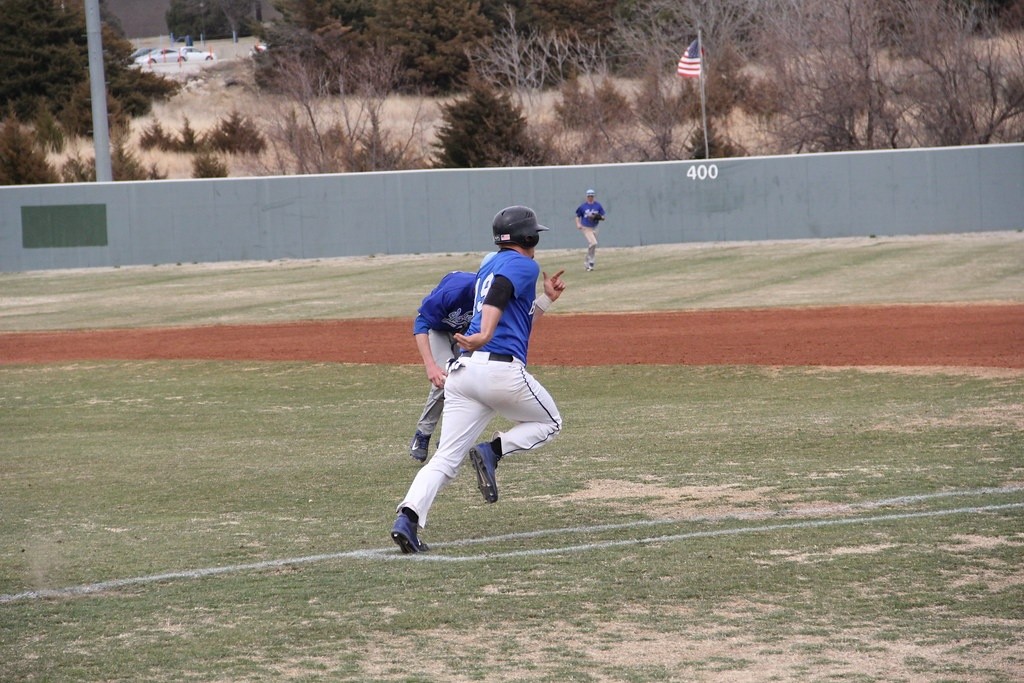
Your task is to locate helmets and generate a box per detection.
[586,190,596,197]
[492,206,549,249]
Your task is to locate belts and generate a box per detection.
[460,350,514,362]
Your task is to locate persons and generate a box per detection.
[392,206,568,551]
[576,190,606,272]
[409,250,477,462]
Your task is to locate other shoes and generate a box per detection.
[589,263,593,270]
[586,268,592,272]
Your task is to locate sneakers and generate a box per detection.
[391,514,431,554]
[410,430,432,462]
[469,442,500,504]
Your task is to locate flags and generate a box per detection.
[678,38,704,77]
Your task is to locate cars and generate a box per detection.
[131,46,217,64]
